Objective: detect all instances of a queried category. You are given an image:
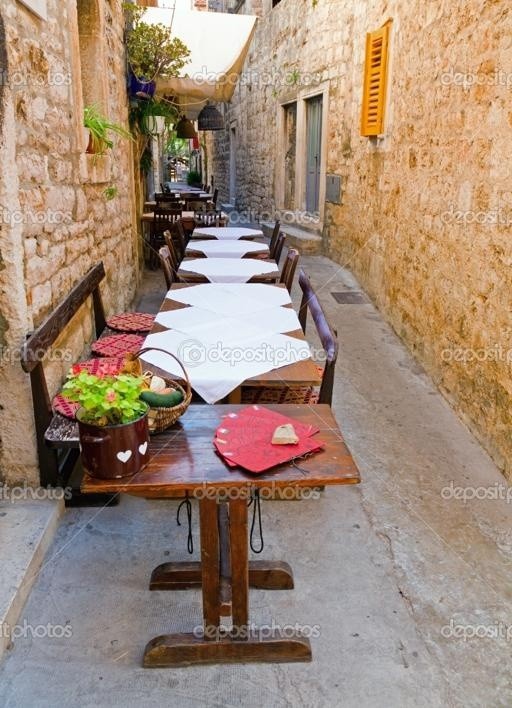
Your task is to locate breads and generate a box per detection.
[270,423,299,445]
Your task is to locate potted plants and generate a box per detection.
[60,367,155,480]
[124,20,190,100]
[80,101,130,156]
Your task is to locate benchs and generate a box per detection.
[291,269,339,406]
[22,260,157,511]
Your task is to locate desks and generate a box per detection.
[59,397,363,669]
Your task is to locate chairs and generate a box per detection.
[137,177,230,237]
[279,247,301,289]
[156,229,178,283]
[268,223,288,258]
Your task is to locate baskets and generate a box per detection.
[126,347,192,435]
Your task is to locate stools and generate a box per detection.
[90,309,156,362]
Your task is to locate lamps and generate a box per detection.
[198,100,227,131]
[175,113,201,139]
